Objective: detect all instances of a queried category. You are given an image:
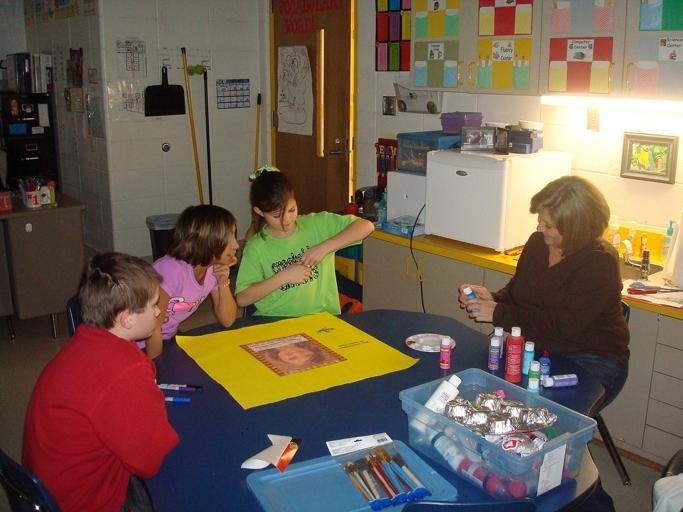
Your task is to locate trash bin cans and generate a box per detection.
[145,212,181,263]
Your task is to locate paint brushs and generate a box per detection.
[345,447,426,501]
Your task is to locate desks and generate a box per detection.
[129,309,601,512]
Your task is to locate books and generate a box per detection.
[4,51,55,93]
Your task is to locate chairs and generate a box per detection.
[652,447,683,511]
[0,451,61,511]
[402,498,539,512]
[66,294,87,336]
[585,302,632,486]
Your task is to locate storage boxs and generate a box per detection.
[440,111,483,136]
[398,365,597,503]
[397,130,460,175]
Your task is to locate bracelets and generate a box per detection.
[215,279,232,287]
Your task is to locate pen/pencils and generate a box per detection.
[17,176,41,192]
[157,382,201,404]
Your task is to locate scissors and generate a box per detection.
[629,281,676,290]
[627,288,683,294]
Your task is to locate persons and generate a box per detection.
[267,337,327,373]
[133,202,239,352]
[233,170,377,320]
[20,252,181,512]
[458,176,631,423]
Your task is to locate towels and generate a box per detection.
[650,471,683,512]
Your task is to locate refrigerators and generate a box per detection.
[423,148,570,252]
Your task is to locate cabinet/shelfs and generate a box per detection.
[362,235,483,333]
[405,0,539,97]
[0,89,63,194]
[640,302,683,466]
[0,193,90,339]
[535,0,682,101]
[483,260,660,456]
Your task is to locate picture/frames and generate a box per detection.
[460,125,497,151]
[620,132,679,185]
